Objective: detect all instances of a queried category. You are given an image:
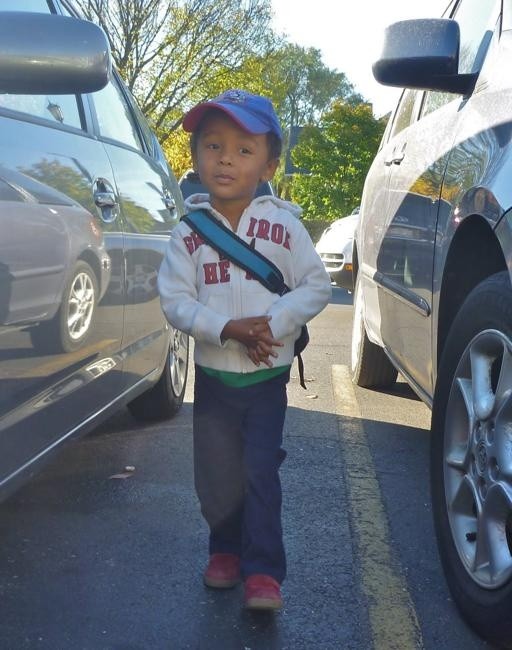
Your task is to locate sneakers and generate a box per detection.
[204,551,242,590]
[242,573,284,610]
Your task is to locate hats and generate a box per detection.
[182,90,284,142]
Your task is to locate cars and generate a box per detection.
[362,186,445,282]
[104,232,172,304]
[349,0,512,650]
[0,0,190,506]
[0,166,112,353]
[315,213,359,291]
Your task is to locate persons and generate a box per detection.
[157,88,333,611]
